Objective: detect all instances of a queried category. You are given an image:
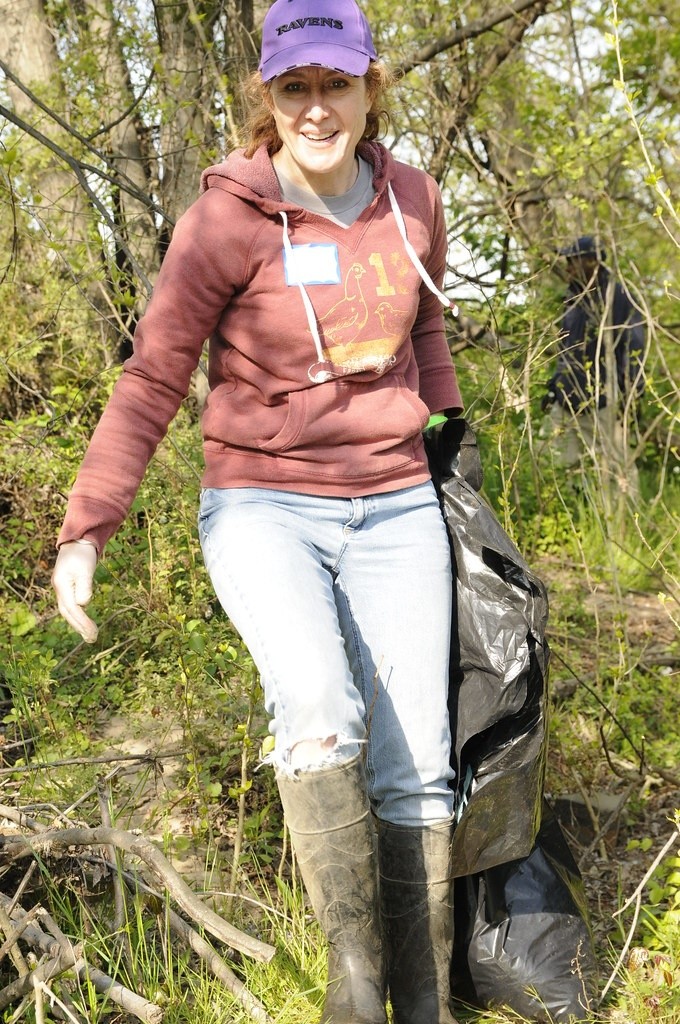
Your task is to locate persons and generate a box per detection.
[50,0,487,1024]
[540,236,645,585]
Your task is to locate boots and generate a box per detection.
[369,804,459,1024]
[274,743,390,1024]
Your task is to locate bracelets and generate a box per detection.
[74,539,96,548]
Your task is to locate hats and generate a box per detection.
[555,236,604,256]
[258,0,377,84]
